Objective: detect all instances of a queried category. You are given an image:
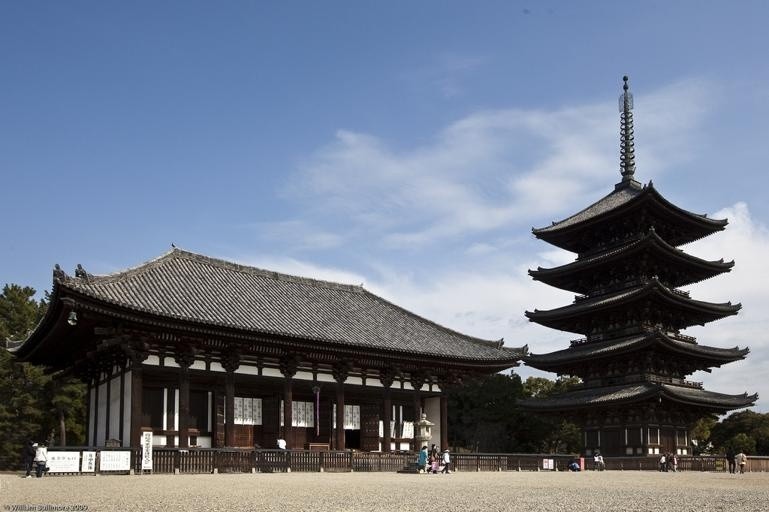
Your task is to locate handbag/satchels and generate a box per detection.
[432,462,439,470]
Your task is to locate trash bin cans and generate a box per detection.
[580,458,585,471]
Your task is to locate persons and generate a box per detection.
[441,450,451,474]
[569,461,582,471]
[427,444,438,473]
[276,435,287,451]
[33,443,49,472]
[24,438,36,476]
[659,454,668,473]
[737,450,747,475]
[593,453,605,468]
[671,454,678,470]
[415,445,428,474]
[729,445,738,473]
